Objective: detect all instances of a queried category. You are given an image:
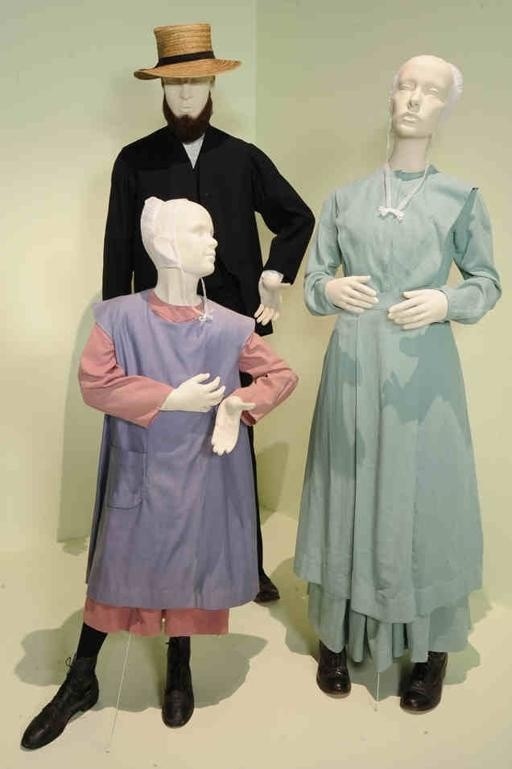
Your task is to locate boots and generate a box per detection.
[20,652,101,751]
[160,636,194,728]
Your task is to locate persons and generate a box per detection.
[294,51,503,713]
[82,17,317,607]
[20,193,298,752]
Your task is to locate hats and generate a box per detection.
[132,22,242,81]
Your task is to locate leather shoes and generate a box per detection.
[314,637,351,699]
[420,673,424,676]
[399,650,446,713]
[253,570,280,604]
